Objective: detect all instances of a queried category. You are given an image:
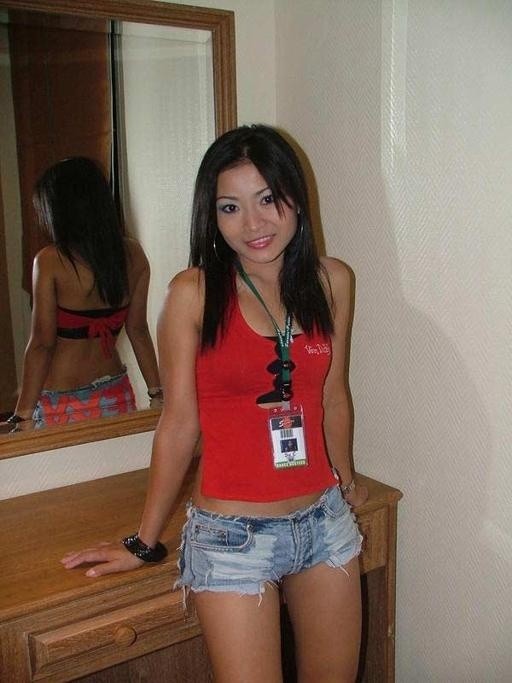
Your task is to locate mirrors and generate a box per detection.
[0,1,240,459]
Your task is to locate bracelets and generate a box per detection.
[338,472,359,499]
[146,385,163,397]
[119,530,171,566]
[6,414,27,426]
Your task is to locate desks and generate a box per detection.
[0,455,403,683]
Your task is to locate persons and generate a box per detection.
[1,155,164,436]
[57,124,371,682]
